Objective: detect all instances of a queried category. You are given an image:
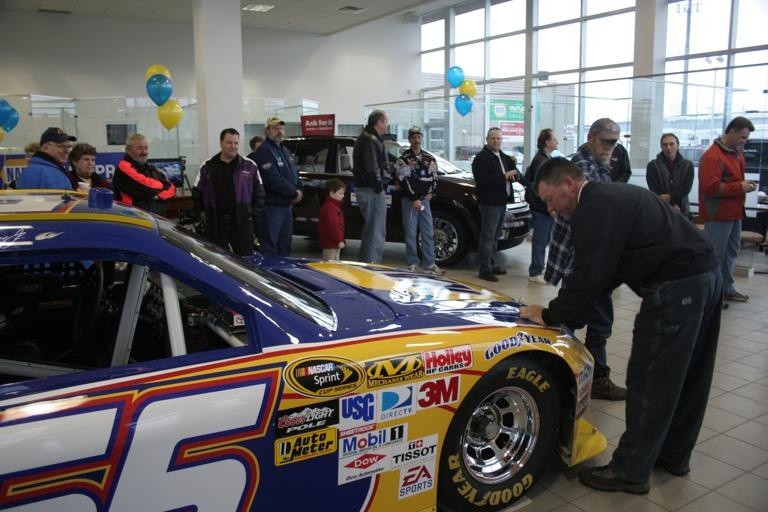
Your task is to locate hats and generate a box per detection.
[407,125,426,139]
[265,116,287,128]
[41,126,78,147]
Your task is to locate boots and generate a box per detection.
[591,366,629,401]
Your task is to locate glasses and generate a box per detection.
[25,153,33,157]
[46,142,76,150]
[270,127,284,130]
[594,133,617,146]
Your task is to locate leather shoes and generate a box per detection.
[479,273,500,283]
[493,267,508,276]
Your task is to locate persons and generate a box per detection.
[525,130,559,284]
[520,157,724,494]
[68,144,111,193]
[610,144,632,183]
[354,110,391,264]
[472,128,519,281]
[113,134,176,214]
[699,117,757,310]
[544,119,627,400]
[251,137,265,151]
[246,116,303,256]
[646,134,694,220]
[192,129,266,255]
[318,178,345,261]
[15,127,73,190]
[395,127,446,274]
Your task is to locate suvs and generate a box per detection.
[282,136,533,267]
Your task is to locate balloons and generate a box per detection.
[0,99,11,126]
[455,93,473,116]
[0,127,6,141]
[145,65,171,84]
[447,67,465,88]
[158,99,182,130]
[459,80,478,98]
[146,74,174,106]
[2,107,19,132]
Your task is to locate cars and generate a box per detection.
[0,187,607,512]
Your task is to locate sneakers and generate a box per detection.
[422,265,446,276]
[722,301,729,311]
[657,451,691,476]
[726,290,750,303]
[410,265,417,271]
[528,276,548,285]
[578,461,651,496]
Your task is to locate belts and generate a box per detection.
[219,213,235,219]
